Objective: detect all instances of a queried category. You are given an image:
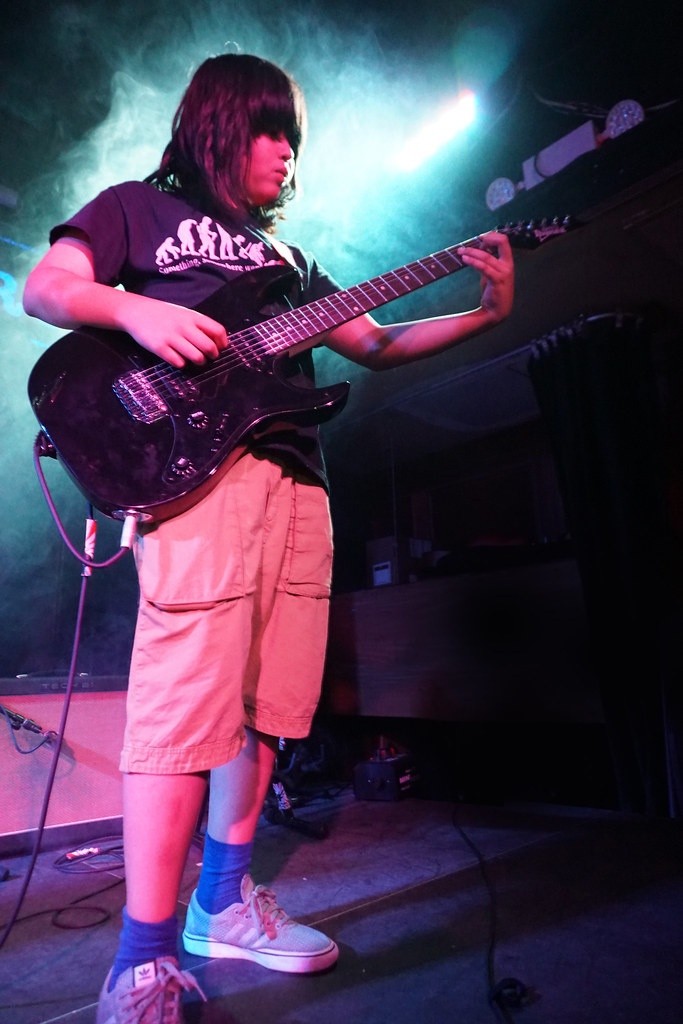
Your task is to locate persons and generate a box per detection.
[21,54,514,1024]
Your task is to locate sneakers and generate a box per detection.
[97,955,207,1024]
[182,873,339,973]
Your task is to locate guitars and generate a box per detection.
[24,209,574,533]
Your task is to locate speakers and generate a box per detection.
[0,675,128,854]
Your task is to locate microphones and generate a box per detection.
[43,731,58,743]
